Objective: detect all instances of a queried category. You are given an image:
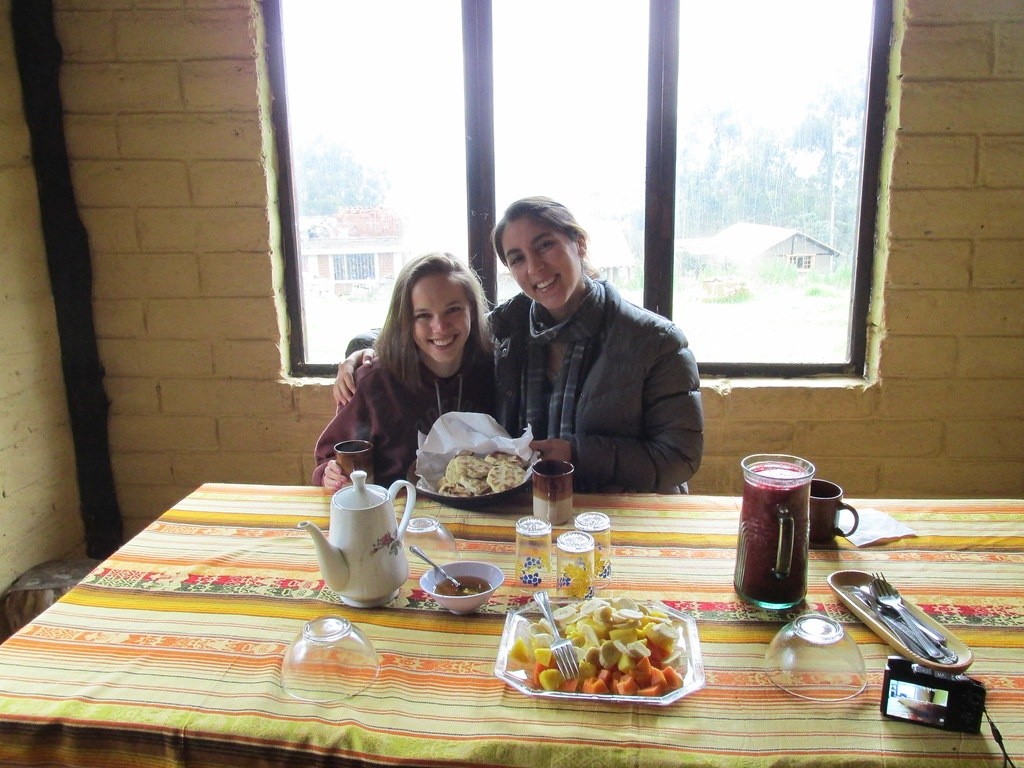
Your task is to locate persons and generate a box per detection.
[333,197,704,497]
[313,253,493,491]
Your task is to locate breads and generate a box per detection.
[436,450,527,498]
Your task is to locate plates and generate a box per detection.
[493,598,706,707]
[827,570,973,674]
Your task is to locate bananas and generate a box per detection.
[527,596,684,670]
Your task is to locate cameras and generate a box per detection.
[879,655,986,734]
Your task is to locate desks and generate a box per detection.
[0,483,1024,768]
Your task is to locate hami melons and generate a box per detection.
[533,638,684,697]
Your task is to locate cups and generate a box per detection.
[575,512,612,589]
[531,459,575,525]
[333,440,374,480]
[556,531,595,599]
[809,479,859,543]
[515,515,553,586]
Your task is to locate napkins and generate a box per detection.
[836,506,916,547]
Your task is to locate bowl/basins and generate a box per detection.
[419,560,504,615]
[415,454,539,506]
[401,515,460,576]
[764,613,868,701]
[281,614,380,702]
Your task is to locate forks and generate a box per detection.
[869,572,945,658]
[533,590,580,681]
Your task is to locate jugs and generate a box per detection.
[734,452,815,609]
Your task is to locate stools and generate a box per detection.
[4,556,101,635]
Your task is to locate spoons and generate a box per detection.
[837,578,958,665]
[410,545,479,596]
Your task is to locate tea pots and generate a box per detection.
[297,470,417,607]
